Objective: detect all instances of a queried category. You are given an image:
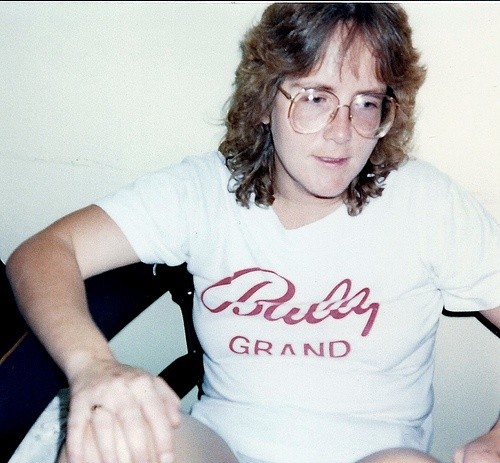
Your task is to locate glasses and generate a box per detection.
[278,85,398,139]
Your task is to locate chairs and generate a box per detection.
[0,261,499,461]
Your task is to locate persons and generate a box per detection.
[0,0,500,463]
